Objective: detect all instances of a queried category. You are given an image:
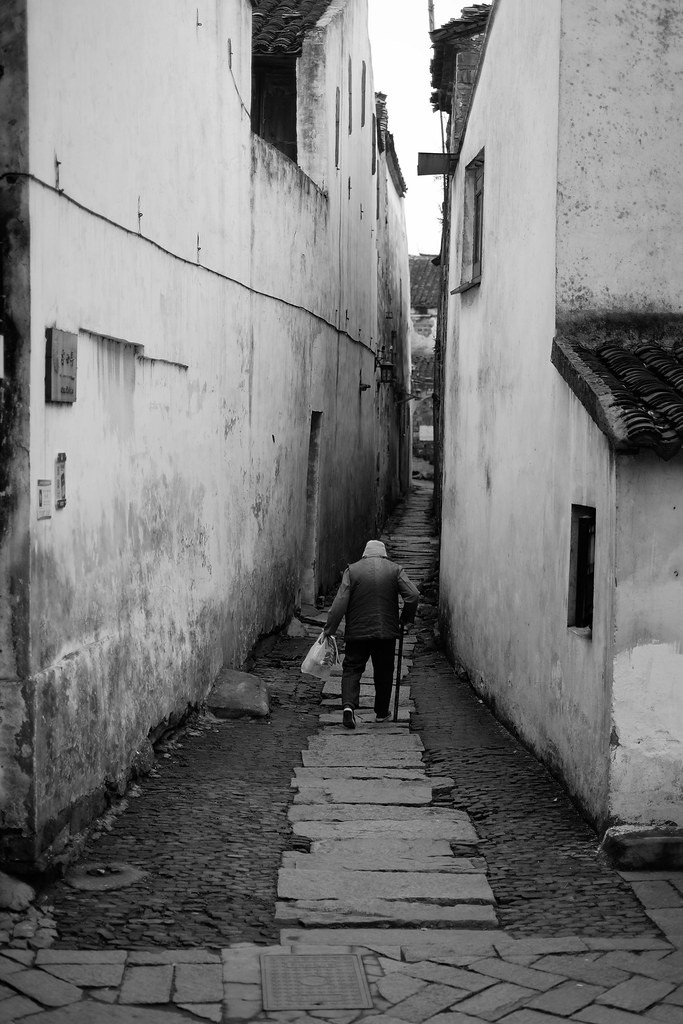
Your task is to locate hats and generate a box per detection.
[362,540,387,557]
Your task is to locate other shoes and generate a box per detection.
[376,710,391,721]
[343,705,356,729]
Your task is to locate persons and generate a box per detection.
[323,540,420,730]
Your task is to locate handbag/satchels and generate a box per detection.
[330,635,340,666]
[301,632,335,678]
